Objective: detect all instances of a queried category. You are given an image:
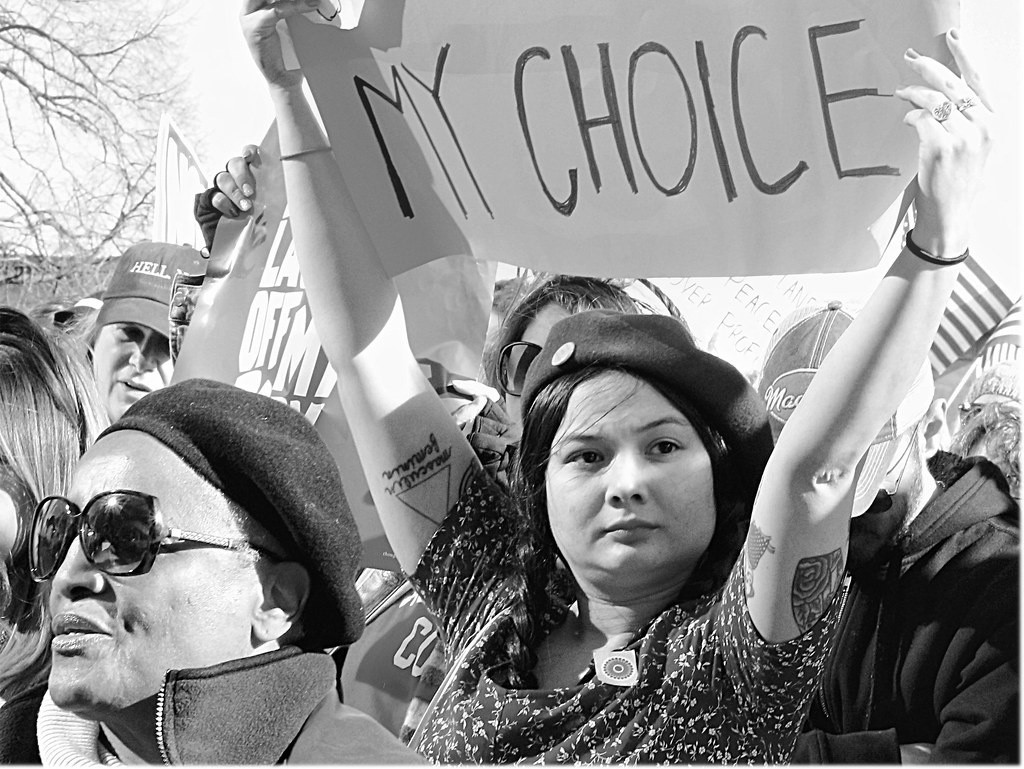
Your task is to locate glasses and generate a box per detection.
[29,489,283,583]
[497,340,543,400]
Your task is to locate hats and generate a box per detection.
[966,362,1019,408]
[96,382,364,647]
[523,309,773,511]
[54,291,105,324]
[759,303,934,516]
[94,240,207,343]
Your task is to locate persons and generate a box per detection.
[241,0,997,766]
[0,304,113,764]
[757,297,1021,767]
[29,241,1021,498]
[26,376,431,767]
